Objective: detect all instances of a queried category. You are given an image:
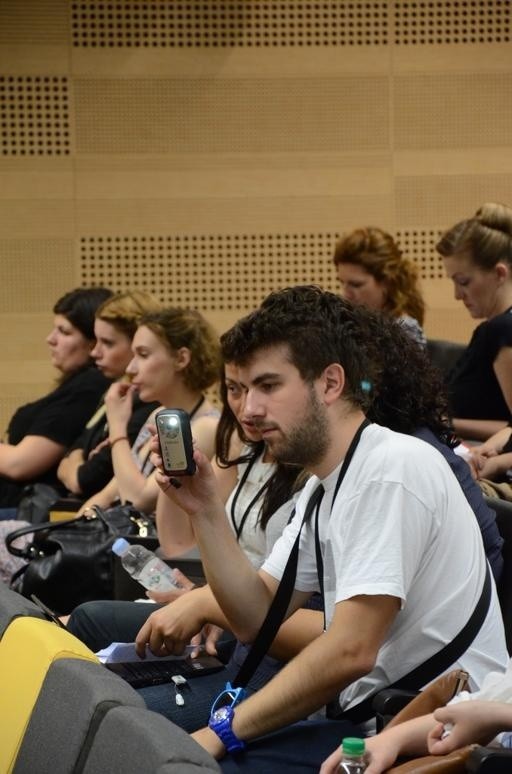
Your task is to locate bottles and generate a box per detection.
[332,737,368,774]
[111,538,181,592]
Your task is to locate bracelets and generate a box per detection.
[110,436,130,448]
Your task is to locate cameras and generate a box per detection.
[156,408,196,476]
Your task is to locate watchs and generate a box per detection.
[207,704,243,755]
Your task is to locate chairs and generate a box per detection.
[10,657,146,774]
[1,581,47,640]
[80,706,221,773]
[0,615,101,774]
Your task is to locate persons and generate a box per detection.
[23,308,248,652]
[331,228,428,353]
[436,208,512,439]
[1,285,111,519]
[318,653,512,772]
[138,308,324,732]
[1,291,163,580]
[469,410,512,480]
[363,310,503,585]
[188,288,512,772]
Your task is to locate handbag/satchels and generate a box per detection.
[378,669,490,773]
[20,498,161,616]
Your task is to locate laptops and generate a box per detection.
[29,594,226,688]
